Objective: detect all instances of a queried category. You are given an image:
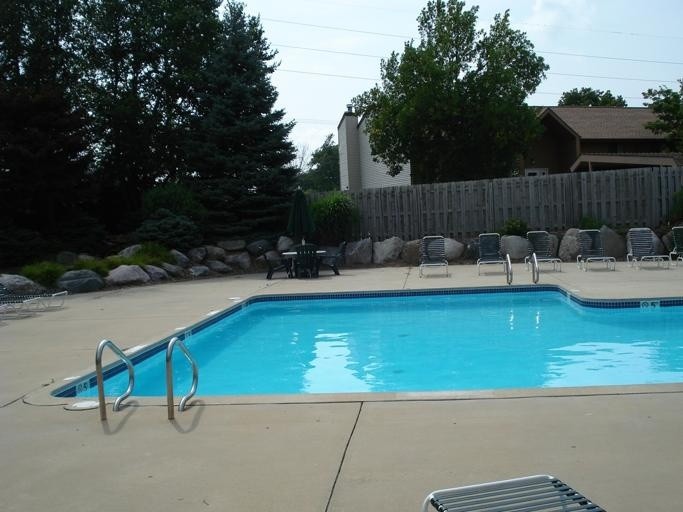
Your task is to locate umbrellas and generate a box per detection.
[286,185,316,252]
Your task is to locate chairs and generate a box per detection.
[421,474,606,512]
[257,242,347,280]
[0,283,68,326]
[419,227,683,278]
